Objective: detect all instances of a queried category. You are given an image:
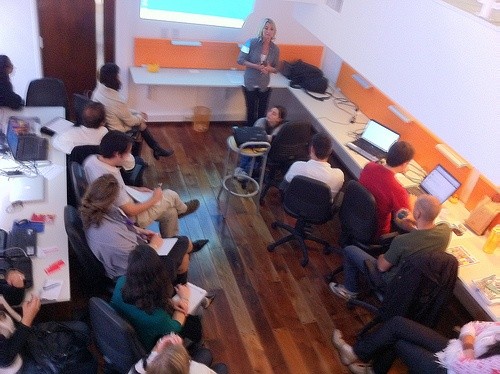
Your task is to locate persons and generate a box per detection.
[0,55,23,110]
[332,319,500,374]
[84,130,199,237]
[359,140,415,245]
[110,244,202,354]
[79,173,209,296]
[238,108,287,173]
[127,333,227,374]
[236,16,279,125]
[279,133,345,201]
[329,195,450,299]
[90,63,172,160]
[0,270,98,374]
[53,101,145,186]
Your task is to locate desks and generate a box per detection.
[130,65,500,323]
[0,106,70,308]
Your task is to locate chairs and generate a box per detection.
[266,175,344,268]
[322,179,399,285]
[347,251,460,342]
[258,118,313,205]
[26,77,149,374]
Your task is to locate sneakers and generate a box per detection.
[329,282,358,301]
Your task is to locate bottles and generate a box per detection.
[484,224,500,254]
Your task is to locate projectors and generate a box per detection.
[233,126,271,150]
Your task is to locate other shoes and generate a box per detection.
[333,329,359,364]
[238,171,248,181]
[135,158,148,168]
[153,148,173,158]
[177,199,200,218]
[349,361,374,374]
[233,166,244,178]
[189,240,209,254]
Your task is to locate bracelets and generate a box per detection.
[463,343,473,350]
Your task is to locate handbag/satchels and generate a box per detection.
[300,72,328,94]
[233,126,269,149]
[288,63,323,81]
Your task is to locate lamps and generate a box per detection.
[353,74,373,90]
[437,144,468,167]
[387,105,412,124]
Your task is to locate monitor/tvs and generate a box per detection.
[6,116,48,160]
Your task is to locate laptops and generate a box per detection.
[9,174,43,203]
[0,228,37,257]
[347,119,400,162]
[408,164,462,204]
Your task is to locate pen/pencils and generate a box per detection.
[43,282,60,291]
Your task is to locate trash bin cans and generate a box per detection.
[192,106,210,132]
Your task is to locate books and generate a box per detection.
[156,238,178,255]
[171,282,207,313]
[472,275,500,305]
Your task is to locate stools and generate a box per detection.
[217,136,271,221]
